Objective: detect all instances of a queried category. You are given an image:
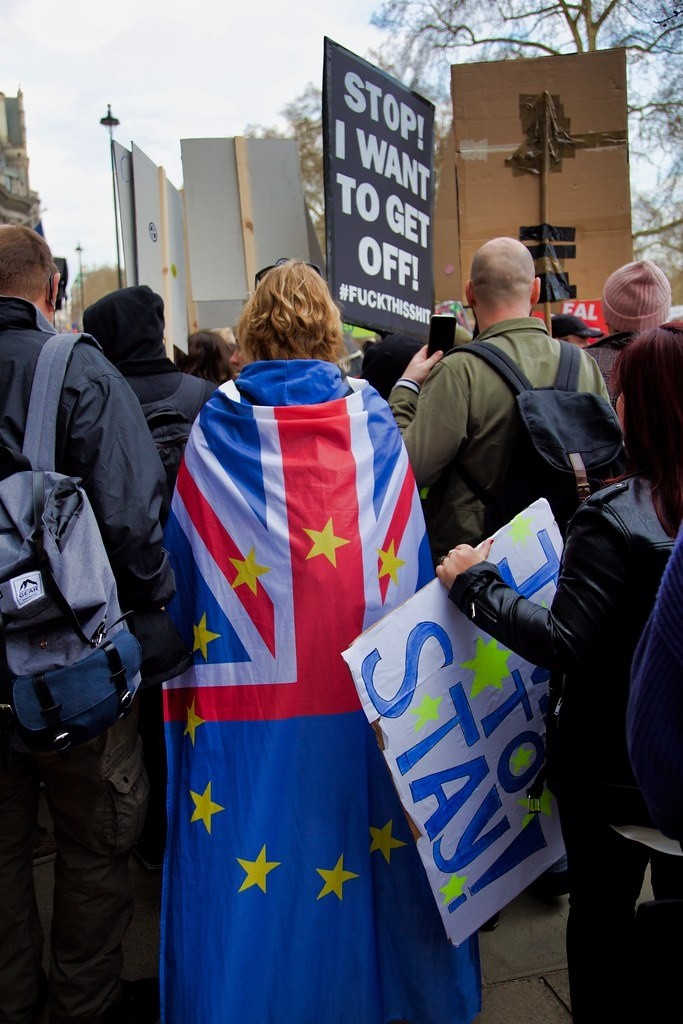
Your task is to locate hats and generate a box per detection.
[362,339,423,390]
[602,253,672,330]
[548,312,604,342]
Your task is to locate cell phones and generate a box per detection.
[426,314,457,371]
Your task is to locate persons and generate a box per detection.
[0,224,683,1024]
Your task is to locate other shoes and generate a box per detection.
[30,830,58,868]
[529,872,573,896]
[112,974,161,1024]
[132,840,167,870]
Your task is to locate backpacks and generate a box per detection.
[431,340,627,543]
[0,333,142,748]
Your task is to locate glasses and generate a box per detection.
[255,262,323,291]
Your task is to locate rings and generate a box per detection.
[438,556,446,564]
